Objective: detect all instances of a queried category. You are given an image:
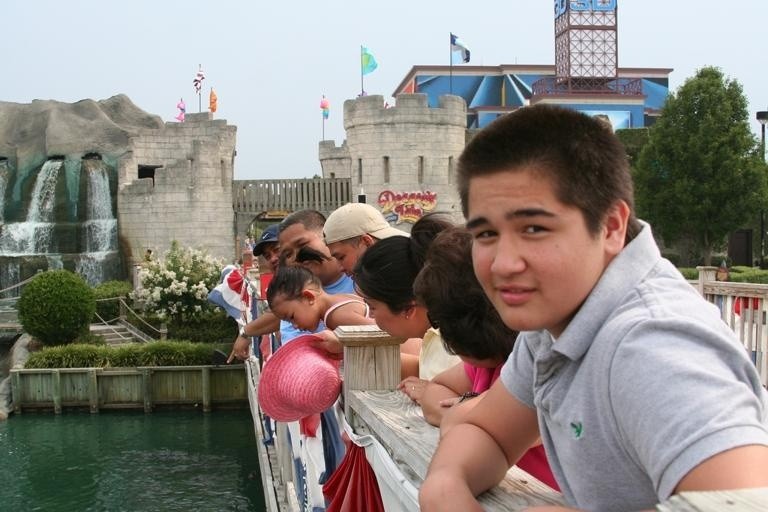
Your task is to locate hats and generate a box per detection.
[252,223,282,257]
[255,334,343,425]
[321,201,413,245]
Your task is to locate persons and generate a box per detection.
[321,202,409,279]
[414,224,562,512]
[278,209,354,341]
[307,216,463,403]
[226,222,281,364]
[418,105,768,511]
[267,247,378,332]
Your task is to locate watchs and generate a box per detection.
[239,327,248,339]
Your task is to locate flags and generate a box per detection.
[449,31,470,64]
[359,46,378,75]
[208,82,218,112]
[193,63,208,112]
[319,94,330,119]
[175,98,187,122]
